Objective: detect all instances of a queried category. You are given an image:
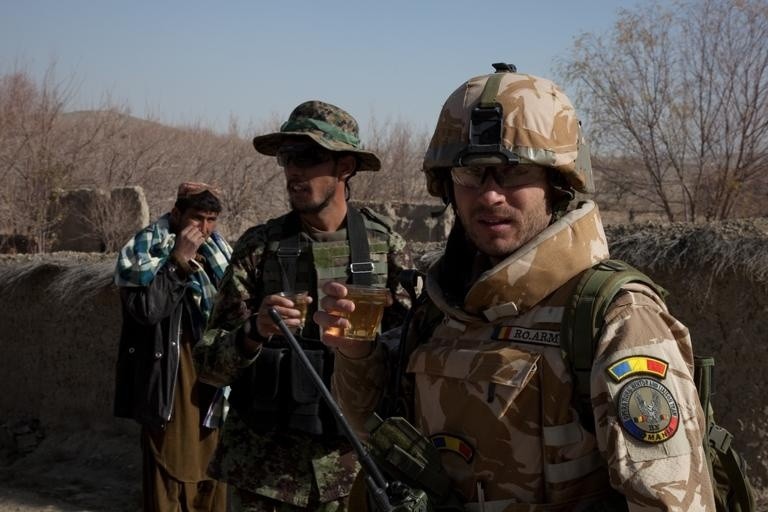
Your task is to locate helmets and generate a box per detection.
[422,63,595,196]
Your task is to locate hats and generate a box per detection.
[254,102,381,171]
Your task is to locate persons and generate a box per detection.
[113,181,236,512]
[312,71,717,512]
[191,100,423,512]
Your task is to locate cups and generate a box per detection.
[278,290,307,329]
[339,284,390,343]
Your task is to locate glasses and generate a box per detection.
[277,144,330,166]
[450,166,541,188]
